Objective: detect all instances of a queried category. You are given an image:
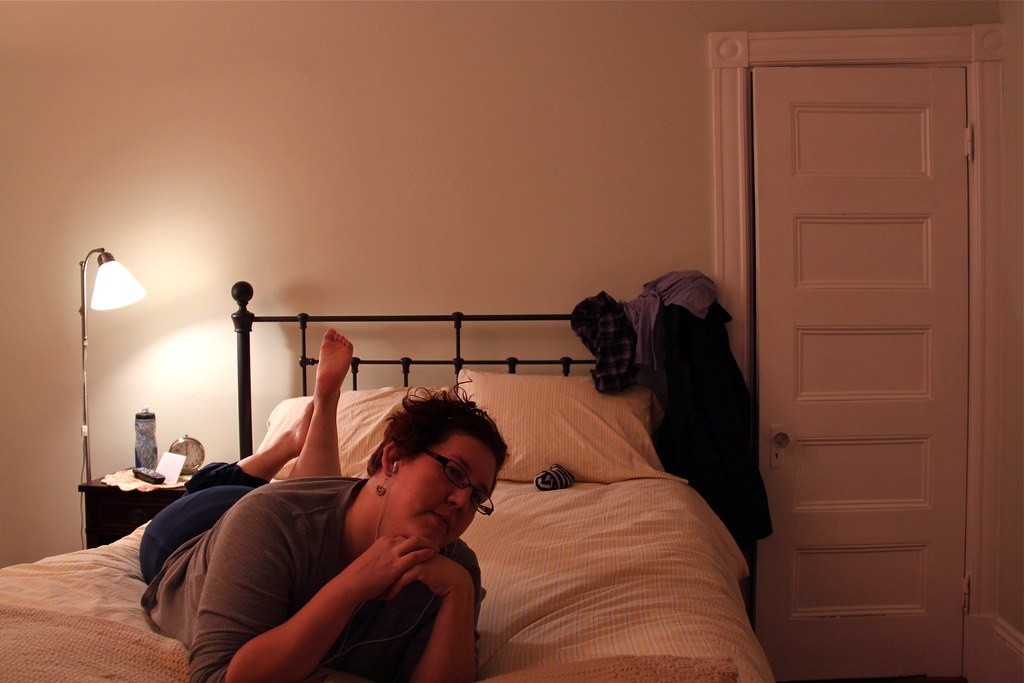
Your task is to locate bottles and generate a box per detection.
[135,406,158,471]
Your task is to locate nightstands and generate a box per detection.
[79,468,197,550]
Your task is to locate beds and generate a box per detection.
[0,281,780,683]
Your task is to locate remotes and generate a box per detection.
[133,467,165,484]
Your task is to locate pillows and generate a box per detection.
[458,367,693,488]
[257,386,460,482]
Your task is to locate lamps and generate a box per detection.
[78,246,147,483]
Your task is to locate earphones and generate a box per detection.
[393,461,397,473]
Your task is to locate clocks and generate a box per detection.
[169,434,206,477]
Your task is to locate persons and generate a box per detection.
[139,328,511,683]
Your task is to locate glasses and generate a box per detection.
[420,447,494,516]
